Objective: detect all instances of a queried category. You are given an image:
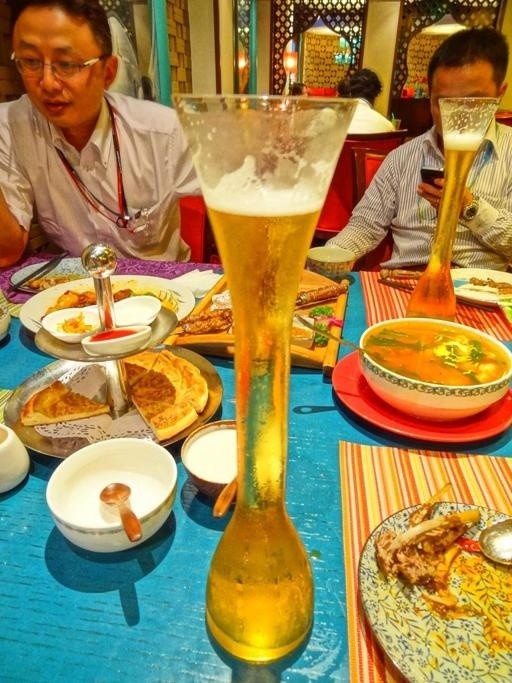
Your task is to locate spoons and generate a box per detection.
[101,482,142,542]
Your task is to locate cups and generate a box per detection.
[405,99,499,320]
[163,95,358,662]
[392,118,401,130]
[1,423,30,496]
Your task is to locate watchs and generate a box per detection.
[463,193,479,222]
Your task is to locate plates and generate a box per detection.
[44,308,102,345]
[445,266,512,304]
[35,306,177,358]
[353,500,511,682]
[20,275,195,336]
[8,347,223,460]
[84,325,154,358]
[11,256,91,290]
[331,349,509,446]
[112,296,161,328]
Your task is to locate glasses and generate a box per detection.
[8,50,105,79]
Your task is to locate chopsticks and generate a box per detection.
[378,278,499,313]
[9,247,71,291]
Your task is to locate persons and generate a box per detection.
[0,0,205,269]
[337,69,395,134]
[292,83,308,97]
[325,27,512,272]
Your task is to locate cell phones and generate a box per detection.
[420,167,445,189]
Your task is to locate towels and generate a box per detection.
[359,269,510,341]
[0,254,224,319]
[339,440,511,682]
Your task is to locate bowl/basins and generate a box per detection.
[306,245,355,280]
[358,315,511,421]
[180,419,236,506]
[46,436,178,554]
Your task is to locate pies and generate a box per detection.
[20,380,110,427]
[123,350,209,441]
